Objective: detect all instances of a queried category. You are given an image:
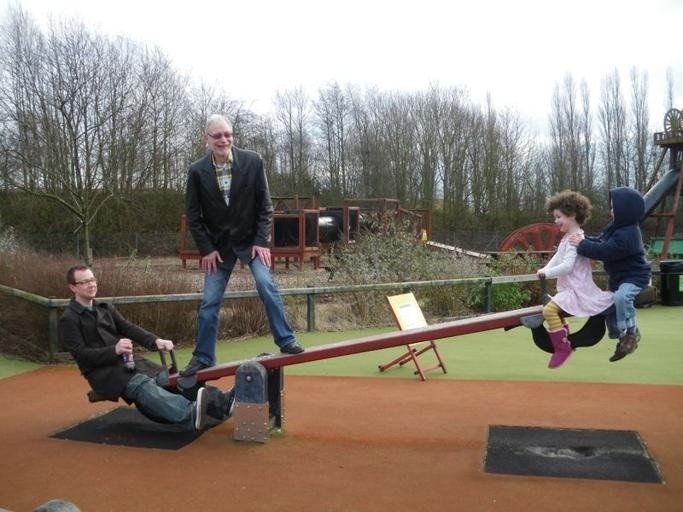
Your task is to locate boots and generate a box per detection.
[547,326,572,370]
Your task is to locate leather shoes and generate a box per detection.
[282,341,303,355]
[180,357,208,377]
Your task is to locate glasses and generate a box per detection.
[74,278,97,285]
[207,132,233,138]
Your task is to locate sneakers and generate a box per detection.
[609,326,640,361]
[225,386,237,419]
[194,388,208,434]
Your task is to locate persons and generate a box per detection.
[176,113,303,378]
[534,188,616,368]
[59,266,235,431]
[567,185,652,362]
